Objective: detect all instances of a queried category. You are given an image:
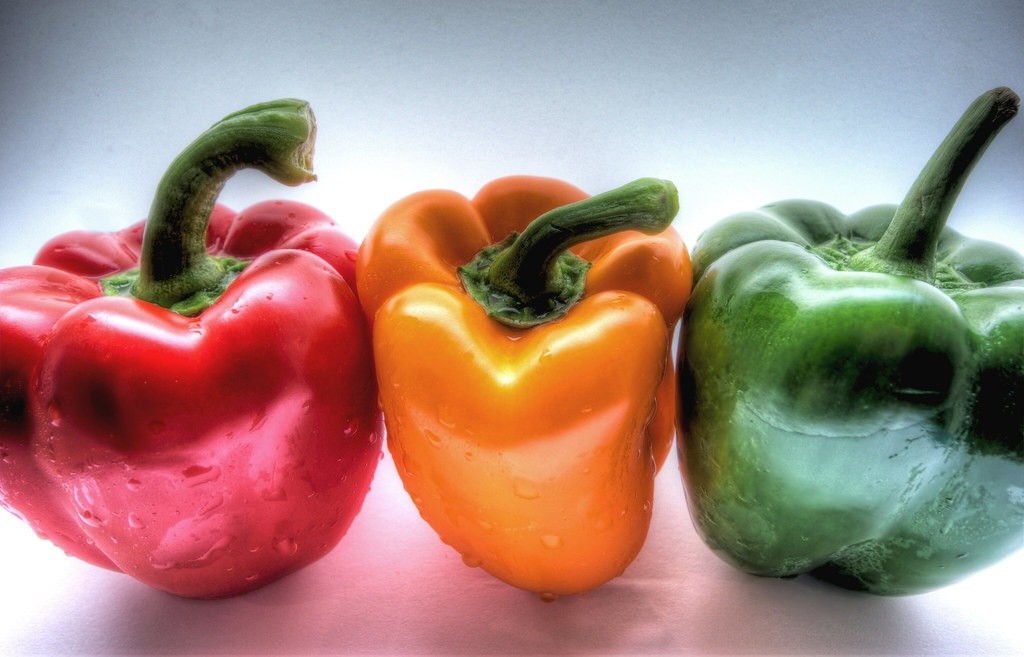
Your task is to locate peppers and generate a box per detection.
[675,89,1024,597]
[356,177,692,600]
[2,101,382,602]
